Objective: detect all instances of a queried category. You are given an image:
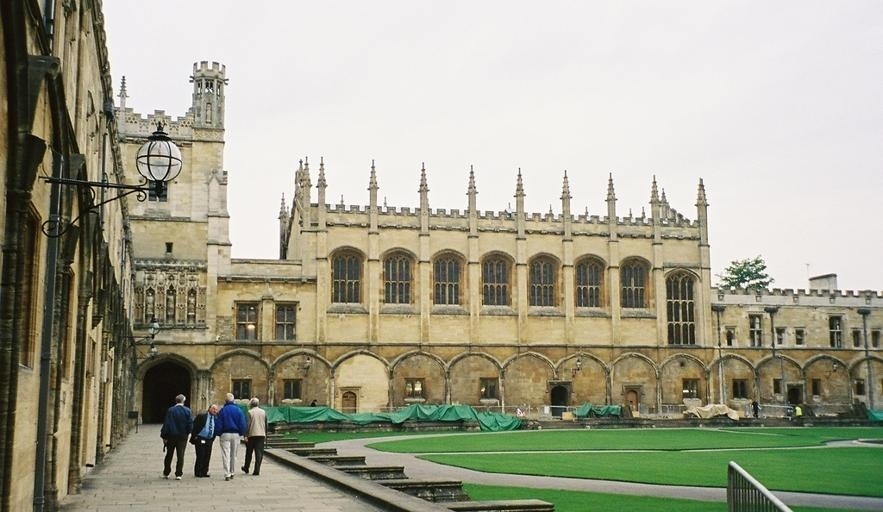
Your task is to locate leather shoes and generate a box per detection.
[203,474,209,477]
[241,467,248,474]
[252,473,258,475]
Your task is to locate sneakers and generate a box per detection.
[176,476,181,480]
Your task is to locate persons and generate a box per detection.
[515,405,525,418]
[794,405,803,419]
[160,394,193,479]
[751,398,761,417]
[190,403,218,477]
[240,397,267,476]
[311,399,316,406]
[214,393,247,480]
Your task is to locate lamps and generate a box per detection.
[572,358,582,370]
[131,342,158,361]
[123,318,160,340]
[38,121,183,238]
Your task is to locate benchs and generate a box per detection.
[727,460,793,512]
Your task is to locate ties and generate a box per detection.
[207,415,212,438]
